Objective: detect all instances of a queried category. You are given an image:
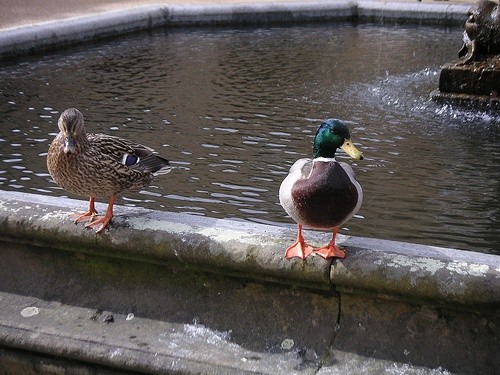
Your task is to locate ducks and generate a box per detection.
[276,117,365,261]
[45,105,173,237]
